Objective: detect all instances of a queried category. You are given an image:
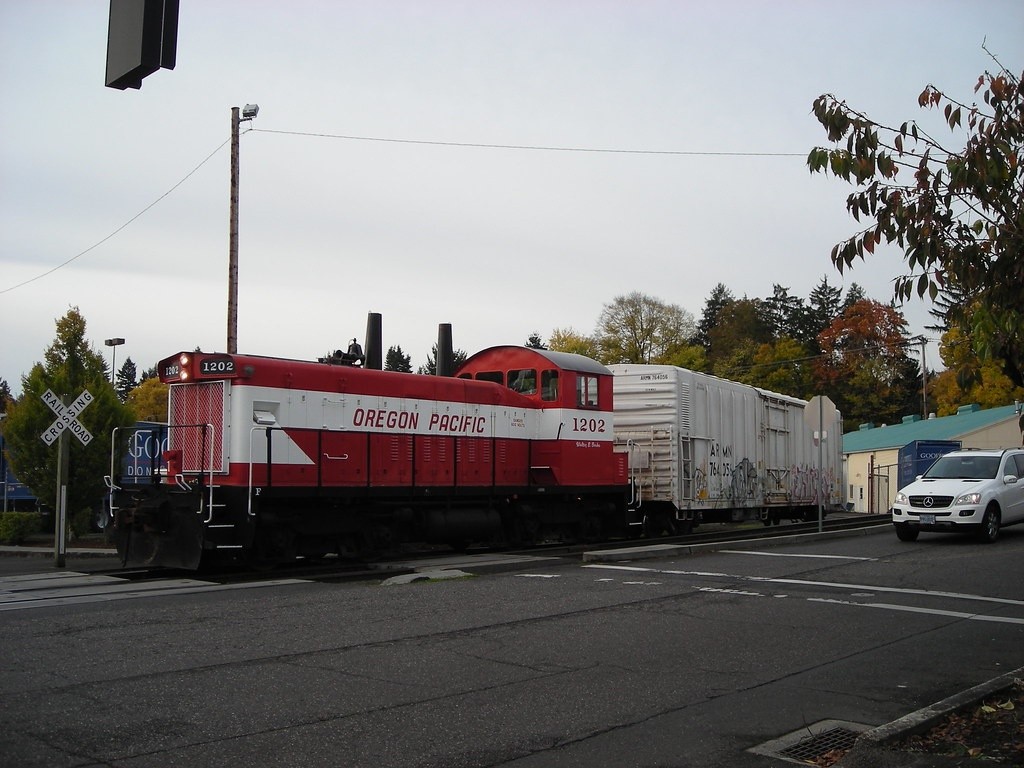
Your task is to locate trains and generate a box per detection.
[99,309,848,571]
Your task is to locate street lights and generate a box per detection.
[226,104,261,354]
[104,338,126,389]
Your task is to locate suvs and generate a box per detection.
[892,446,1024,544]
